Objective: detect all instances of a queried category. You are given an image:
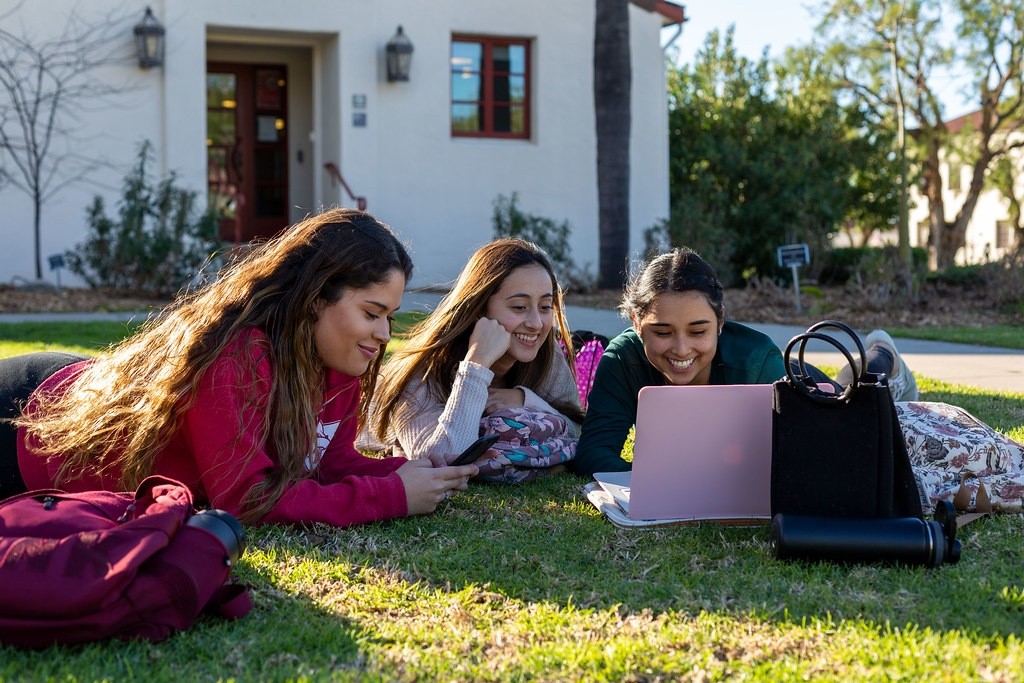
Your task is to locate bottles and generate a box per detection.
[769,516,948,568]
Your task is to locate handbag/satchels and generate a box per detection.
[471,407,578,486]
[556,329,610,411]
[892,401,1024,516]
[770,320,924,520]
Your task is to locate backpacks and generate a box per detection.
[0,475,253,652]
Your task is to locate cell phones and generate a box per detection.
[449,432,502,467]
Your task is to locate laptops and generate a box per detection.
[593,382,835,521]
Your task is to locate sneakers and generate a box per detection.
[865,328,918,401]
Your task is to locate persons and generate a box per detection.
[569,245,919,480]
[0,208,455,514]
[353,237,582,461]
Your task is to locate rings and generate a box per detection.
[439,490,449,503]
